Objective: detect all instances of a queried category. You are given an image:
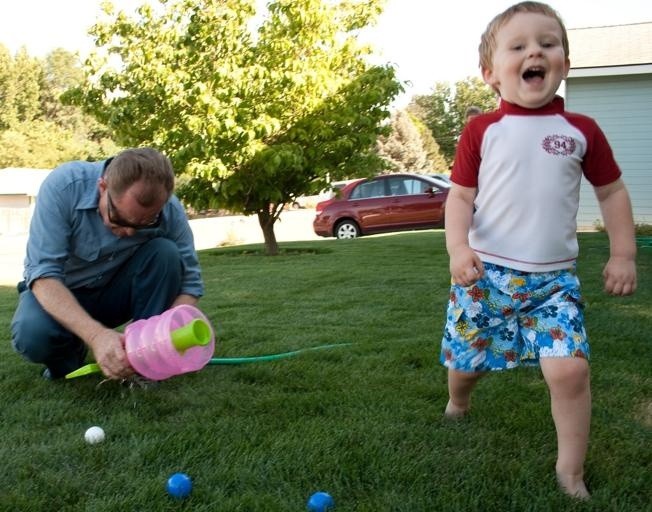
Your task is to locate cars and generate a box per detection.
[314,174,451,238]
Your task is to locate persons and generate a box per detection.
[434,0,640,501]
[8,147,204,384]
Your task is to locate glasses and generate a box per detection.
[107,192,164,230]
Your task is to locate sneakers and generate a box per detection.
[39,360,84,382]
